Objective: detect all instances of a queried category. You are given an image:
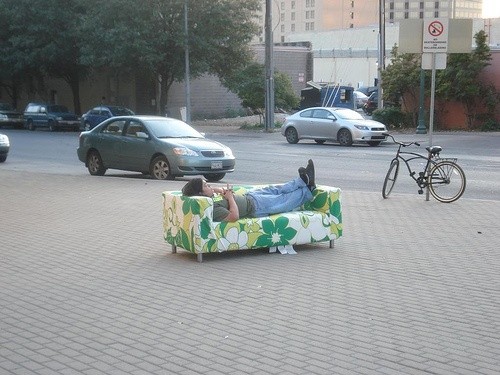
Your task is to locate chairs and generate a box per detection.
[106,125,123,136]
[127,126,145,136]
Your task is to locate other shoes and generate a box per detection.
[304,159,315,186]
[298,167,316,190]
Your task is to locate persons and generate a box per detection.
[181,159,315,222]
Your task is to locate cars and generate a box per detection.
[280,107,389,148]
[77,115,236,182]
[80,106,136,130]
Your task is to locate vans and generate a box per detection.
[22,101,82,133]
[340,92,370,110]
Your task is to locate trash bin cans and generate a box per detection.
[180,107,187,122]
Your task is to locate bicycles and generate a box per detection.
[379,132,467,203]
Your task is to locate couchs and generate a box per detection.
[162,182,343,262]
